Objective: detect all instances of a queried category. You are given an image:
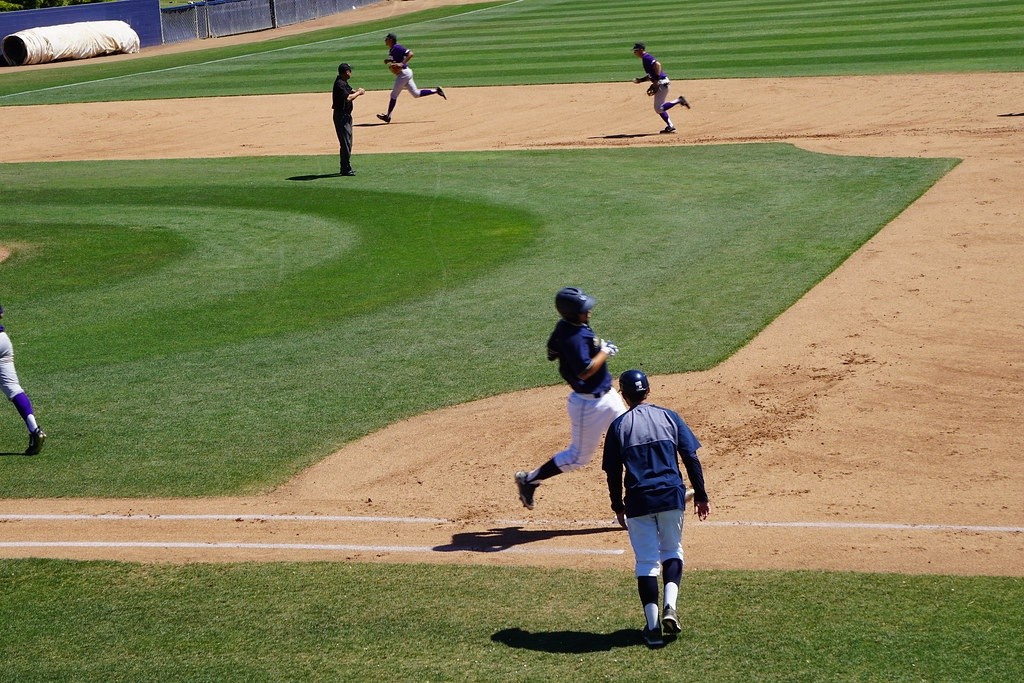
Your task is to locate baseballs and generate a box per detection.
[633,78,638,82]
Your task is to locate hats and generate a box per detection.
[631,43,645,50]
[338,63,354,71]
[384,32,397,39]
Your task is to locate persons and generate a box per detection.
[601,371,710,646]
[332,63,365,176]
[515,288,695,511]
[631,43,690,134]
[376,33,446,122]
[0,302,47,455]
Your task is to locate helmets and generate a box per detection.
[555,288,597,320]
[618,367,650,394]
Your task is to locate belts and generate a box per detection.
[595,385,612,399]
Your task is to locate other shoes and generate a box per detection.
[340,169,354,176]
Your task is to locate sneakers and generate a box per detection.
[661,607,681,633]
[642,623,663,645]
[25,428,47,455]
[377,113,391,122]
[660,126,676,134]
[516,472,540,509]
[436,86,447,100]
[679,96,690,110]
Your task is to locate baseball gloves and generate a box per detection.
[647,83,659,96]
[390,63,403,75]
